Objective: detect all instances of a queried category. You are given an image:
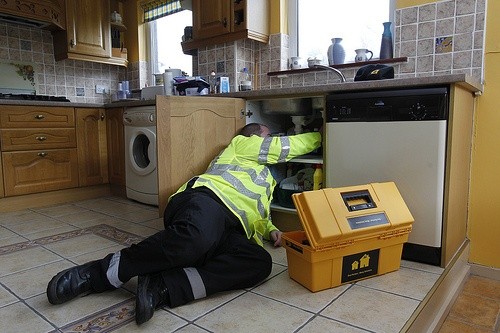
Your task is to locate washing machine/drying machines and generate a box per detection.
[122,105,159,206]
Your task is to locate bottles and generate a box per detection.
[327,38,345,65]
[243,68,250,80]
[164,70,174,96]
[313,165,323,190]
[380,22,392,59]
[209,71,218,94]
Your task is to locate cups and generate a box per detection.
[290,56,301,70]
[240,80,252,91]
[185,88,198,96]
[153,69,182,85]
[307,57,321,68]
[111,90,126,101]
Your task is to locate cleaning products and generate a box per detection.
[209,72,217,95]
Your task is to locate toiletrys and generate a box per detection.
[239,67,252,92]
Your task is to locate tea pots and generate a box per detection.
[111,10,122,23]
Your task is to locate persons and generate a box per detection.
[47,122,323,327]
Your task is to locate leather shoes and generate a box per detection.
[136,274,168,324]
[47,261,95,305]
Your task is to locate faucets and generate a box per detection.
[314,64,347,83]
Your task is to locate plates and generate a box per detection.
[118,98,140,101]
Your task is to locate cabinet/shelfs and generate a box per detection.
[155,84,327,232]
[182,0,270,56]
[0,104,79,199]
[53,0,128,68]
[75,107,109,186]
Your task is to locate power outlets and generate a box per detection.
[95,85,108,94]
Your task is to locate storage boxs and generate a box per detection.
[279,181,415,292]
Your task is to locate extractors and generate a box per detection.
[0,12,52,29]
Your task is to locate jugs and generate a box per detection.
[354,49,373,62]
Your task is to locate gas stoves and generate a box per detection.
[0,92,72,104]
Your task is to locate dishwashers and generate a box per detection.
[324,86,451,266]
